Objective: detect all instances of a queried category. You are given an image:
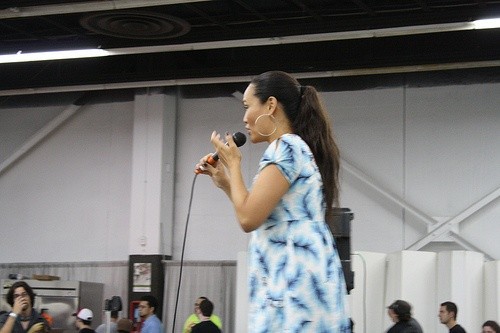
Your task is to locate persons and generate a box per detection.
[0,281,51,333]
[193,71,352,333]
[71,296,500,333]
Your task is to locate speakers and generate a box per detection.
[325,207,354,293]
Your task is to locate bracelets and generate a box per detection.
[8,312,18,319]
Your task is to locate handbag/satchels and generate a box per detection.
[325,208,354,294]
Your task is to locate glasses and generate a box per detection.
[13,292,30,297]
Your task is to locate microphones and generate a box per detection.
[193,131,247,174]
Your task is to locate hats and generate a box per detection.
[388,300,410,313]
[76,308,94,322]
[118,319,132,331]
